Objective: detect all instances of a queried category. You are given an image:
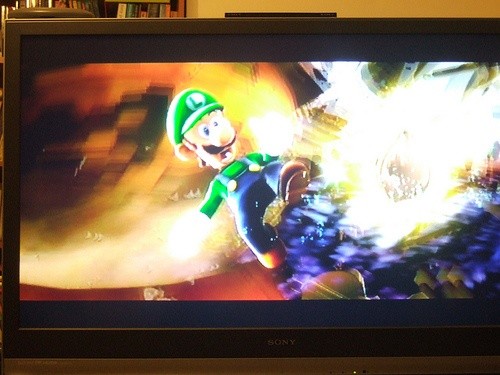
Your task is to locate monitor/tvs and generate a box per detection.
[0,17,500,374]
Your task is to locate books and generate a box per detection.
[0,0,179,59]
